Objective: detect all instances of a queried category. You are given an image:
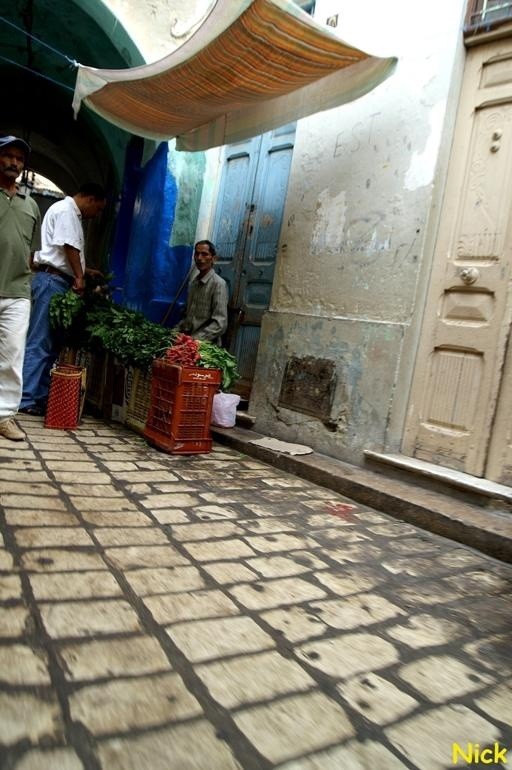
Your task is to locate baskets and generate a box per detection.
[43,366,82,431]
[126,359,220,454]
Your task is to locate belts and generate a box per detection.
[38,264,75,285]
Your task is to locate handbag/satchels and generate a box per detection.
[211,393,240,427]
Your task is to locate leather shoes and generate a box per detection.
[0,416,26,441]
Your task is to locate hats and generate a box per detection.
[0,135,31,153]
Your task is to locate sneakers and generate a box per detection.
[19,406,45,415]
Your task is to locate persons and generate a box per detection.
[0,136,42,441]
[176,240,230,348]
[20,182,106,415]
